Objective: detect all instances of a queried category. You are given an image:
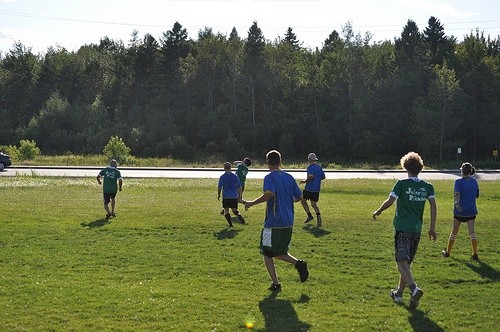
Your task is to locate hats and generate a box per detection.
[308,153,318,161]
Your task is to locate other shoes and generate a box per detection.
[111,212,116,217]
[105,211,111,219]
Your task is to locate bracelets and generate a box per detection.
[454,202,458,206]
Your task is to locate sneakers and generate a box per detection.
[220,210,224,214]
[295,259,309,283]
[243,222,248,225]
[317,215,322,226]
[227,226,234,232]
[409,287,424,310]
[390,288,404,303]
[442,249,451,257]
[472,253,479,260]
[304,216,314,223]
[267,283,281,292]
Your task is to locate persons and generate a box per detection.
[440,162,480,260]
[372,152,437,308]
[242,151,309,290]
[217,162,246,228]
[96,159,123,217]
[219,158,252,214]
[299,153,325,223]
